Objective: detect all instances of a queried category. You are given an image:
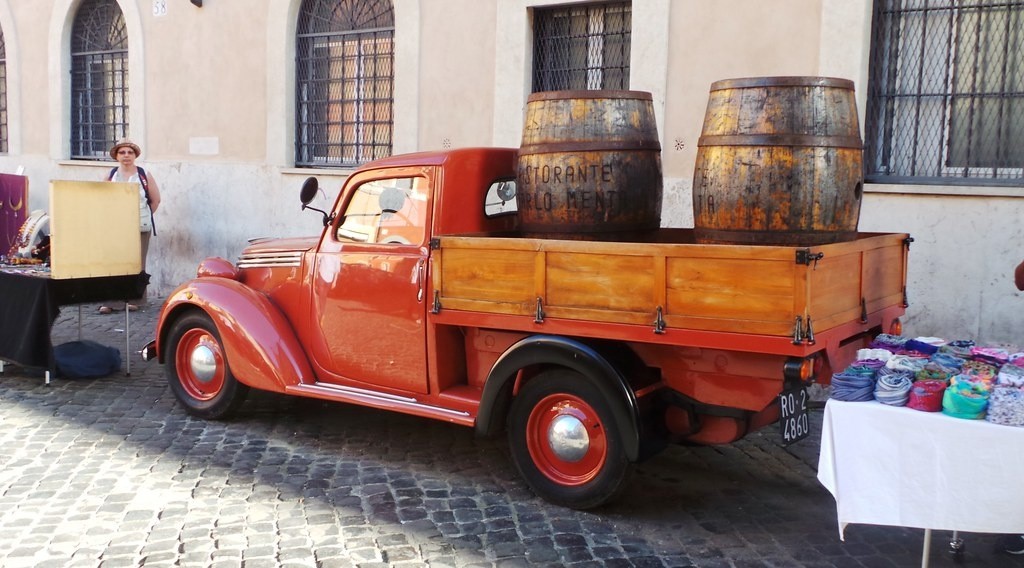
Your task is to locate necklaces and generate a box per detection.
[0,176,22,247]
[17,213,46,248]
[31,234,50,254]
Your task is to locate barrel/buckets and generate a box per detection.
[691,78,864,245]
[516,90,663,244]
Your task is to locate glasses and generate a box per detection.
[116,151,135,155]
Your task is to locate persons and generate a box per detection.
[95,138,161,314]
[1006,262,1024,556]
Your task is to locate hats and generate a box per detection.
[110,138,141,160]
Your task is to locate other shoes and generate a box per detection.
[128,304,139,311]
[99,307,112,314]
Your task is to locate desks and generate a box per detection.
[1,263,150,386]
[815,340,1024,568]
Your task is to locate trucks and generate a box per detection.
[141,147,913,509]
